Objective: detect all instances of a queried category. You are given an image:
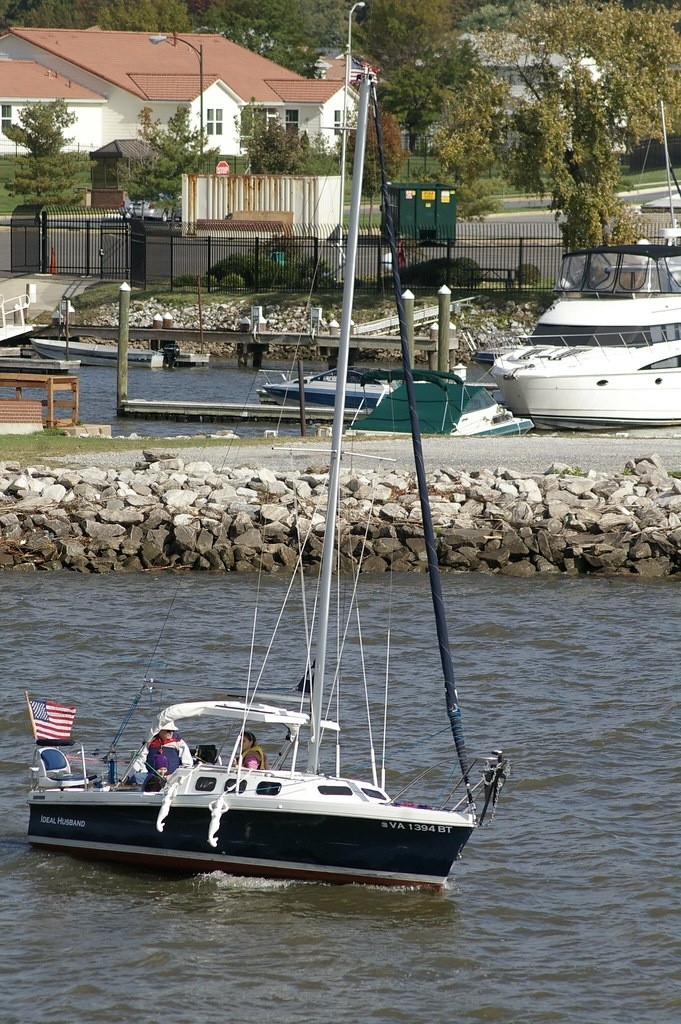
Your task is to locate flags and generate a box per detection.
[29,699,76,739]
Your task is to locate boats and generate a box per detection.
[29,337,164,370]
[255,364,506,411]
[344,368,534,436]
[490,98,681,430]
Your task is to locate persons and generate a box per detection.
[233,731,268,770]
[143,718,194,792]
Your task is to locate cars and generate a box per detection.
[119,191,183,222]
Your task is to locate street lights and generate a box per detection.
[150,34,203,174]
[339,2,365,244]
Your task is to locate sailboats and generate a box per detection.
[25,63,507,897]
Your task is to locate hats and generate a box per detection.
[153,752,168,770]
[162,720,179,731]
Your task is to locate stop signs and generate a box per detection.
[215,161,229,175]
